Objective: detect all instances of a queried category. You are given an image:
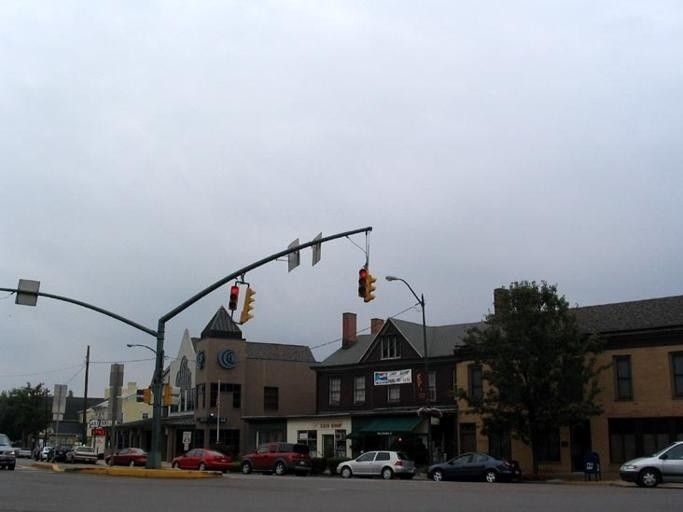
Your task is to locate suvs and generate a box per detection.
[0,433,16,470]
[239,442,312,475]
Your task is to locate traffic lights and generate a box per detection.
[228,286,238,311]
[370,276,377,300]
[168,386,171,405]
[246,289,256,321]
[358,269,367,296]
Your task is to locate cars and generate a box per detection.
[616,440,682,487]
[171,448,232,473]
[103,446,148,468]
[427,451,521,484]
[12,443,98,464]
[335,451,416,479]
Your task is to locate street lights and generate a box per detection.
[124,341,156,356]
[382,273,434,467]
